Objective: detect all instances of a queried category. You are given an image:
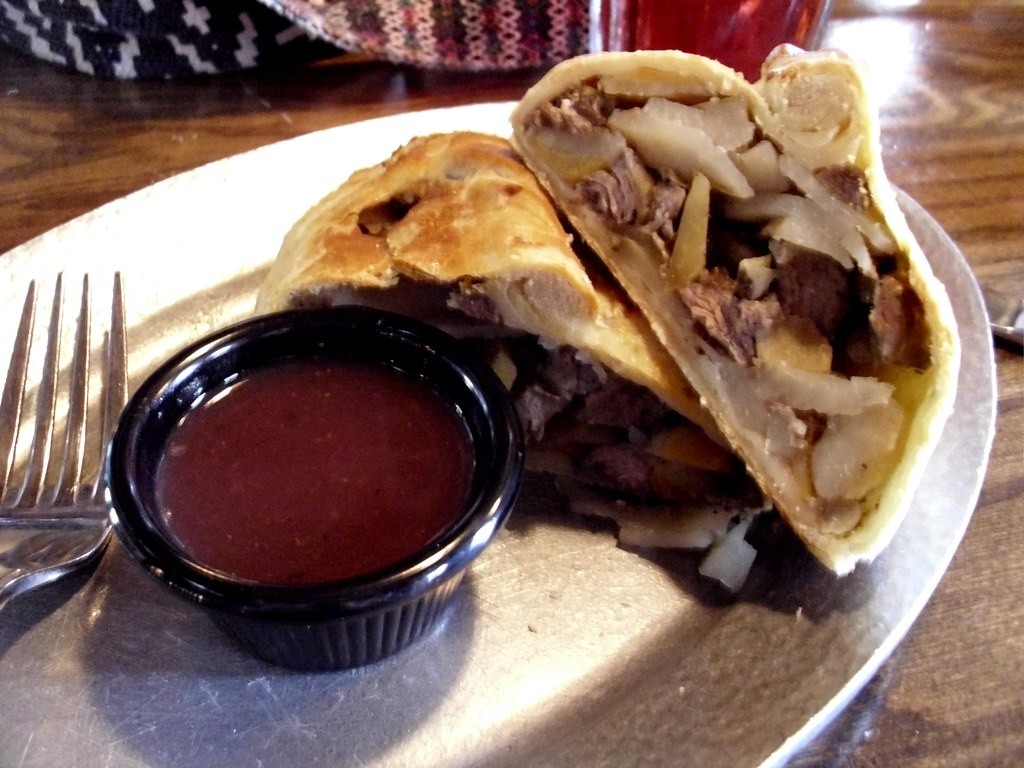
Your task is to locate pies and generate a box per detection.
[248,133,773,590]
[507,43,959,575]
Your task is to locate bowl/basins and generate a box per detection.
[102,304,524,673]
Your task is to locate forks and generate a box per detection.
[1,275,124,618]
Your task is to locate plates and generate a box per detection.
[7,97,996,767]
